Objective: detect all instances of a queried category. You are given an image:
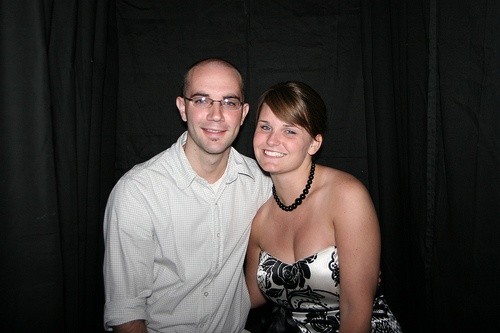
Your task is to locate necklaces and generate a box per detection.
[271,160,315,211]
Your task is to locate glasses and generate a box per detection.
[183,96,243,111]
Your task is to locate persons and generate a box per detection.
[246,80,402,333]
[102,58,383,332]
